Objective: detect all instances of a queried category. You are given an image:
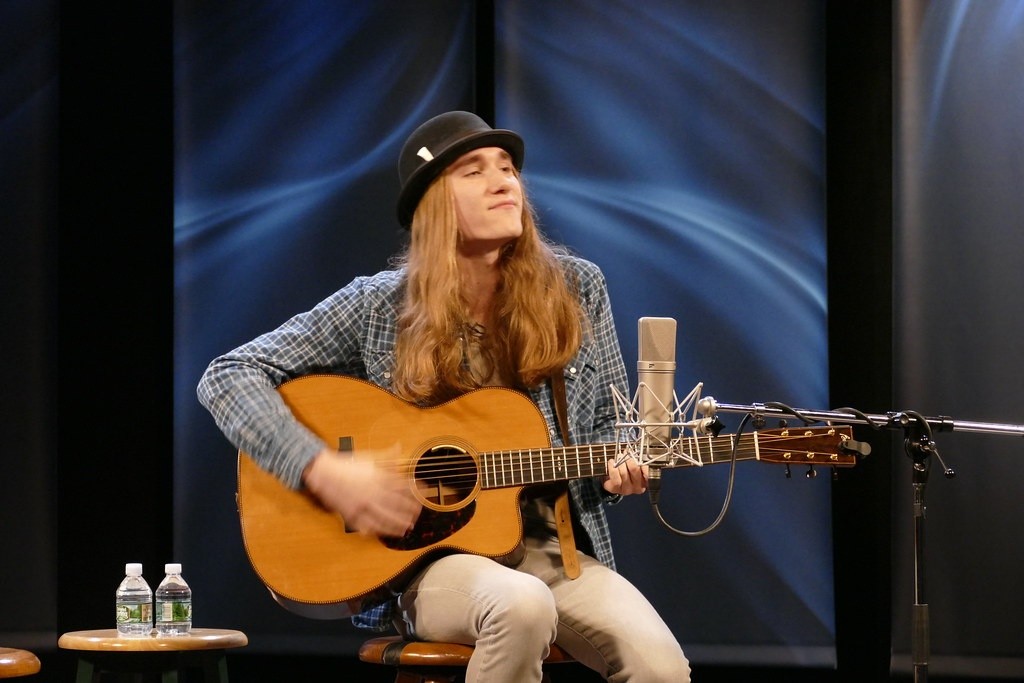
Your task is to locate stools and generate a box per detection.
[358,634,578,683]
[0,644,42,680]
[57,627,246,681]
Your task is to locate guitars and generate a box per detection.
[237,374,872,620]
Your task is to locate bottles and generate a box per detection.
[156,562,192,637]
[117,564,152,637]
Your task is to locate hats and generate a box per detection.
[397,110,524,231]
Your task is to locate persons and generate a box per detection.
[196,109,695,681]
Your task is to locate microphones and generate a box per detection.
[636,316,677,502]
